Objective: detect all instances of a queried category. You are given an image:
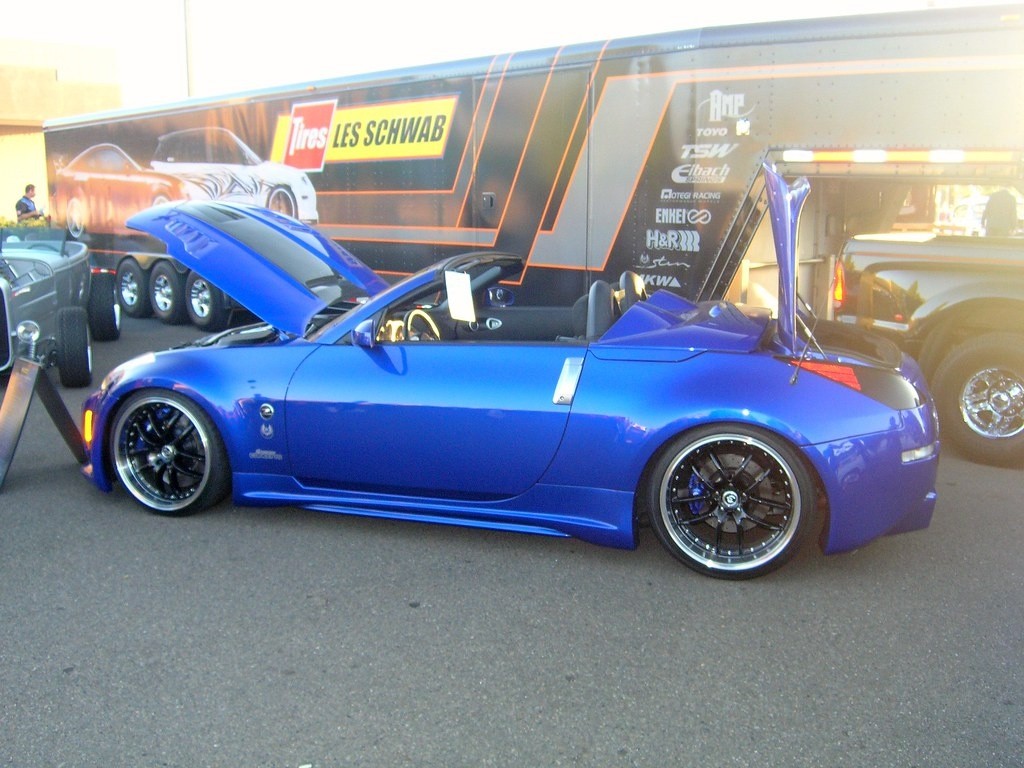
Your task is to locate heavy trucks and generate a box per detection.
[44,5,1024,468]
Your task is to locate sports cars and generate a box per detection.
[81,164,941,582]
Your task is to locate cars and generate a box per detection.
[0,229,124,389]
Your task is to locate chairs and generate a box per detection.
[607,272,646,315]
[574,279,621,339]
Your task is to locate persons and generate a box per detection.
[980,186,1017,235]
[16,184,43,223]
